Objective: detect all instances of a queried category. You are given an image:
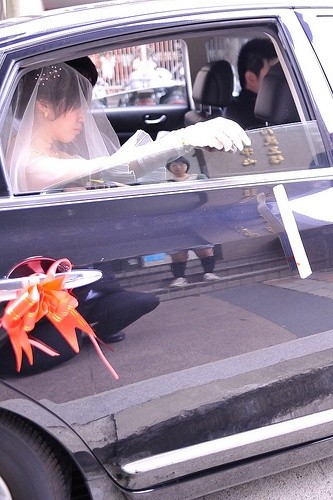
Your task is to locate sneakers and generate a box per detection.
[168,278,188,288]
[202,272,220,281]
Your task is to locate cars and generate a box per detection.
[0,2,333,500]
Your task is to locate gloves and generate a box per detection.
[171,117,254,158]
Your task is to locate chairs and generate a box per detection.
[184,60,234,136]
[246,63,295,131]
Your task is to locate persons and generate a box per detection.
[8,264,160,377]
[164,155,220,288]
[0,56,252,197]
[228,39,280,123]
[100,52,115,85]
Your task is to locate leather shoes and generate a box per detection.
[104,331,125,342]
